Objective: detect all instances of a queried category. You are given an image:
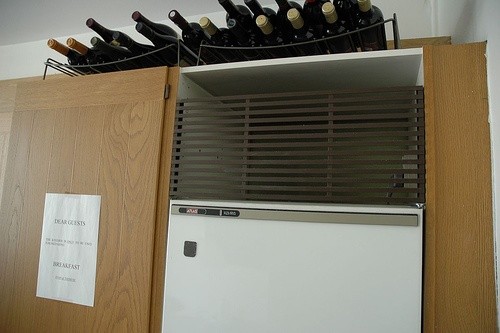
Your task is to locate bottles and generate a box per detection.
[66,38,113,73]
[48,38,97,75]
[354,0,387,52]
[302,0,331,34]
[199,17,246,62]
[90,36,142,72]
[86,18,135,50]
[168,10,221,65]
[112,32,166,68]
[321,2,357,54]
[256,15,297,59]
[131,10,183,41]
[274,0,304,36]
[244,0,279,35]
[227,19,278,61]
[332,0,359,27]
[218,0,261,40]
[135,22,206,68]
[287,8,329,56]
